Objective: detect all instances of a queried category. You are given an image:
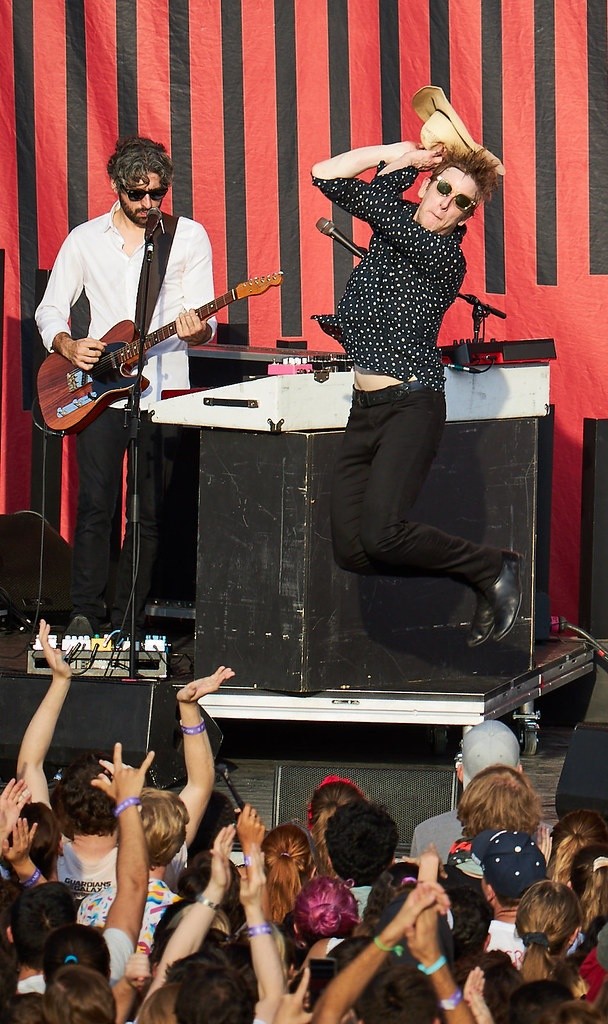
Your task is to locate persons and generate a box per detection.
[309,140,526,647]
[0,620,608,1024]
[33,138,217,649]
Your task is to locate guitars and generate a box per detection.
[37,270,284,435]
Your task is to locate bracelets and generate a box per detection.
[373,936,405,957]
[179,718,205,735]
[195,896,221,911]
[416,955,447,976]
[111,797,141,817]
[247,922,272,938]
[18,867,41,888]
[244,855,256,867]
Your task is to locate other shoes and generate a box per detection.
[63,612,100,639]
[113,627,145,643]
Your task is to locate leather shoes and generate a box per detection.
[488,549,525,641]
[465,590,496,647]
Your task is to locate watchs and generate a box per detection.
[437,988,463,1011]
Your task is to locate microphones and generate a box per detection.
[144,208,162,242]
[316,217,365,258]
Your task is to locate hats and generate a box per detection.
[462,720,521,790]
[469,829,546,898]
[411,86,507,176]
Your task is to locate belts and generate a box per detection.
[352,379,426,407]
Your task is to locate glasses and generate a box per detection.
[121,184,168,201]
[436,176,477,213]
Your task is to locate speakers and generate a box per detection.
[554,722,608,824]
[0,511,72,622]
[0,671,463,859]
